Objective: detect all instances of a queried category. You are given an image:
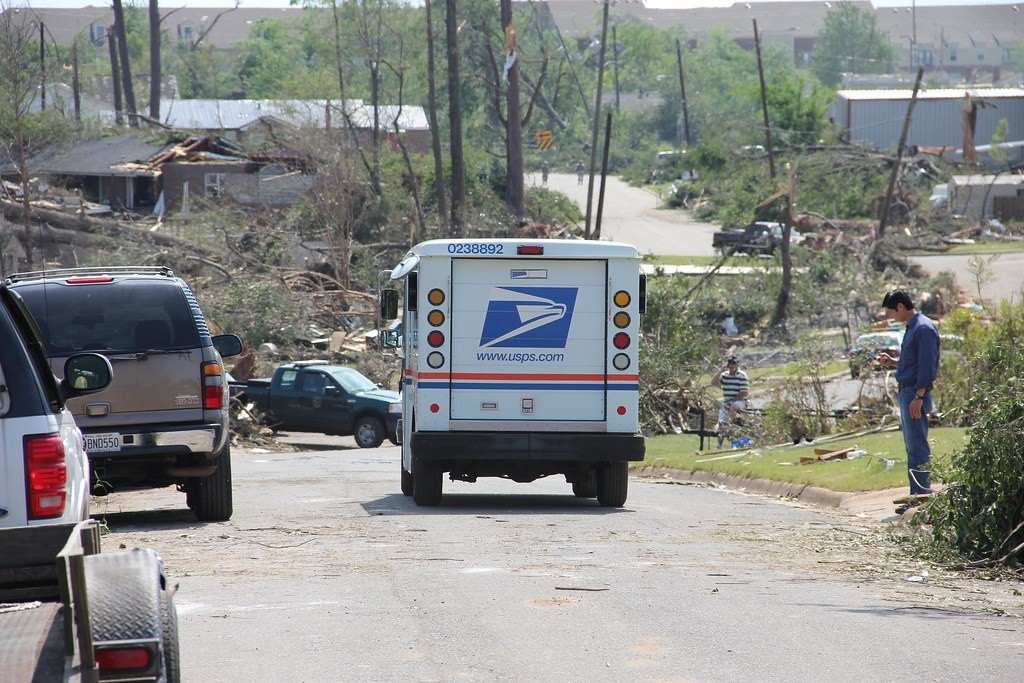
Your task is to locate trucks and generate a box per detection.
[379,238,647,507]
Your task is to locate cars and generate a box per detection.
[850,332,904,378]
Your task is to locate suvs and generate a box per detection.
[6,265,244,521]
[0,281,113,605]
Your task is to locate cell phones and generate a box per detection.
[867,352,881,362]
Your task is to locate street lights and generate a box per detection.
[657,75,696,181]
[752,26,799,178]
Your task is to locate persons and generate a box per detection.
[710,354,749,449]
[576,160,586,186]
[717,301,735,334]
[875,288,941,514]
[541,161,549,186]
[673,175,683,185]
[569,154,577,174]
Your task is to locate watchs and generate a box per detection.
[914,394,923,400]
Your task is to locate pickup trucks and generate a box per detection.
[228,360,402,449]
[712,222,806,256]
